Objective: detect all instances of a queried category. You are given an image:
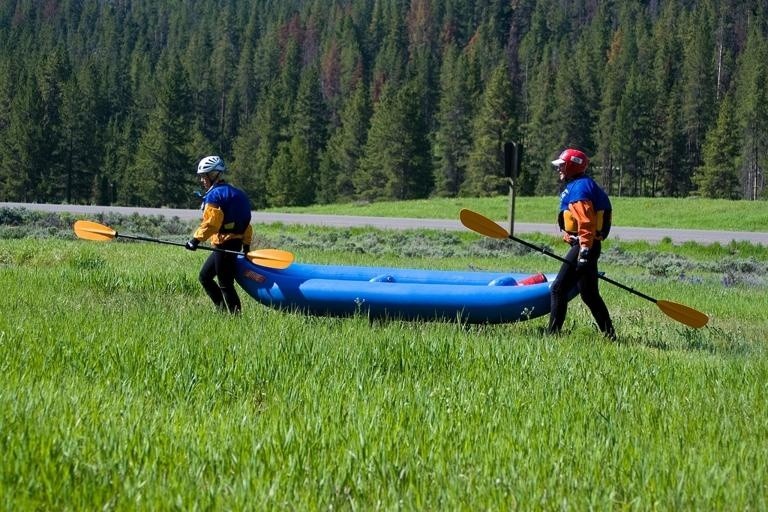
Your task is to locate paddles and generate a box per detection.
[459,208,709,329]
[74,220,294,270]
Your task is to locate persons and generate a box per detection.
[536,149,612,339]
[184,156,253,314]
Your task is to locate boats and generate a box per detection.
[233,252,606,324]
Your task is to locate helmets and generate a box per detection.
[196,155,226,175]
[551,148,590,174]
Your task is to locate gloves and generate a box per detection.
[576,247,589,267]
[185,237,200,251]
